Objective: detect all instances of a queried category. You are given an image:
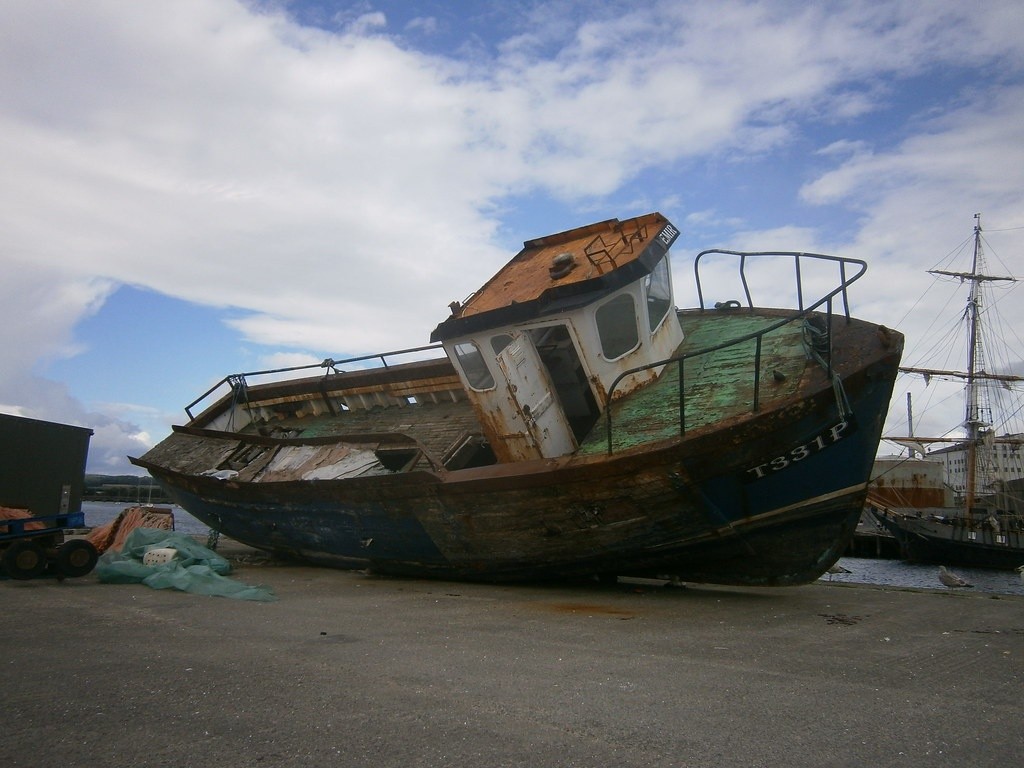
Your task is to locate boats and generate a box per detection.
[122,209,908,592]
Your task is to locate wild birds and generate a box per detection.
[937,565,974,590]
[826,565,852,581]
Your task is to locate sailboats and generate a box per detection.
[858,210,1024,570]
